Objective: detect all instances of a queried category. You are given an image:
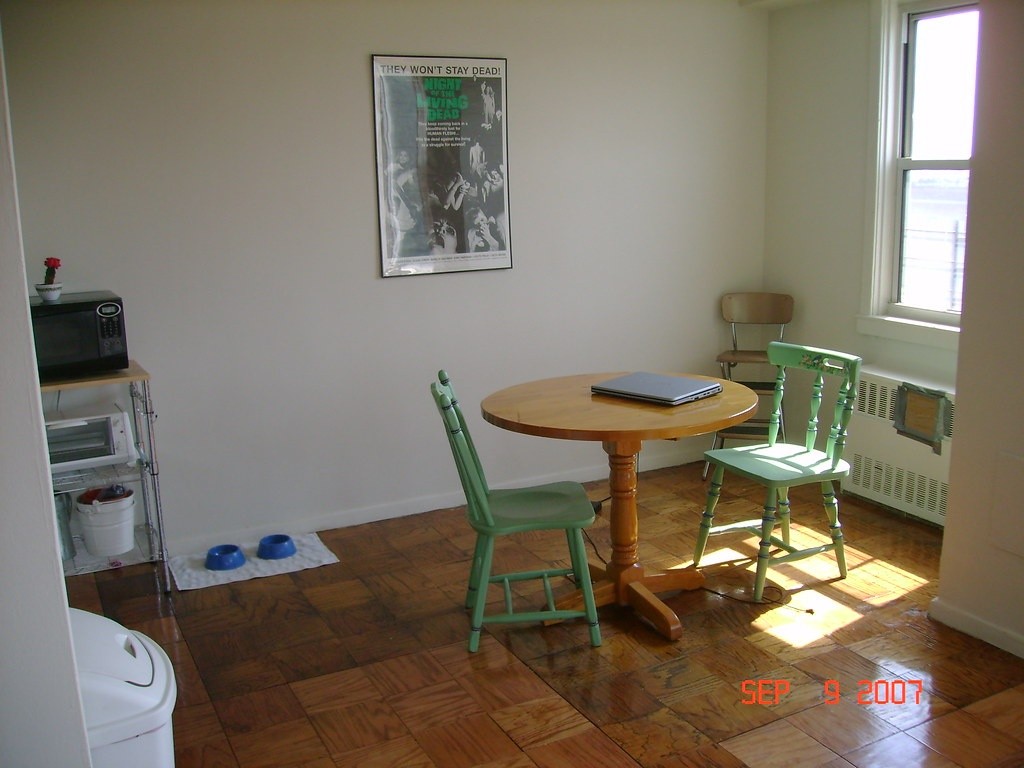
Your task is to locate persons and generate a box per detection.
[383,82,506,257]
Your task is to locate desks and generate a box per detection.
[481,372,760,642]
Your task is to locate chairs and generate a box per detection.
[702,291,795,482]
[429,369,604,654]
[690,340,862,604]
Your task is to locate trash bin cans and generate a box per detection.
[67,607,177,768]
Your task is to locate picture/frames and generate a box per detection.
[370,53,512,279]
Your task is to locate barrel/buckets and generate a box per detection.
[74,488,134,557]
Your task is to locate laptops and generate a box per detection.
[591,370,723,405]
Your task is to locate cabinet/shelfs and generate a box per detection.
[39,358,173,596]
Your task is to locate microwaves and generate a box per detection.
[26,289,130,383]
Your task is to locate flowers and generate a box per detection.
[42,257,61,285]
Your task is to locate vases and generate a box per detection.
[34,284,63,300]
